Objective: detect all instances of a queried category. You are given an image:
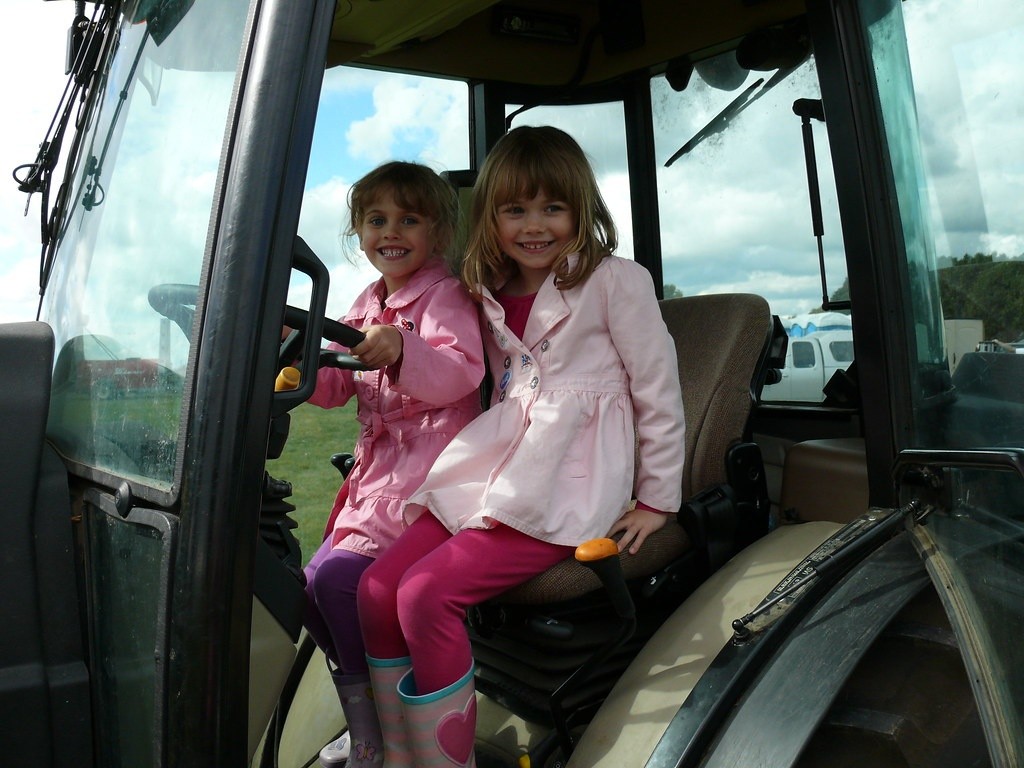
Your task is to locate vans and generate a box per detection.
[759,328,856,405]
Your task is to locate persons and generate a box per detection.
[280,163,484,768]
[356,126,686,767]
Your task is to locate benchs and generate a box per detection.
[467,293,778,724]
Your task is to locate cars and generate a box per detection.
[74,357,179,401]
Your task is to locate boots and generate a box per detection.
[319,730,351,768]
[331,666,385,768]
[397,656,477,768]
[365,652,412,768]
